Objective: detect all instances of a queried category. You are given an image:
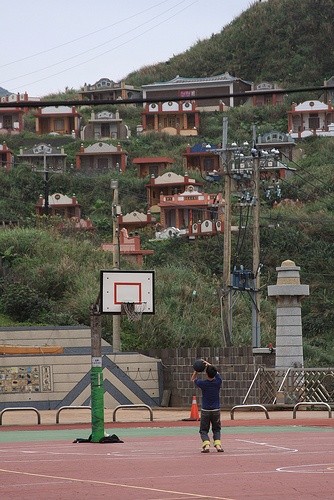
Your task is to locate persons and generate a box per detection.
[191,359,224,453]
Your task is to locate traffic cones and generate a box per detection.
[182,396,201,421]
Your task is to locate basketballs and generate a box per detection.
[194,360,206,372]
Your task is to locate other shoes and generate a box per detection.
[201,449,209,453]
[218,448,224,452]
